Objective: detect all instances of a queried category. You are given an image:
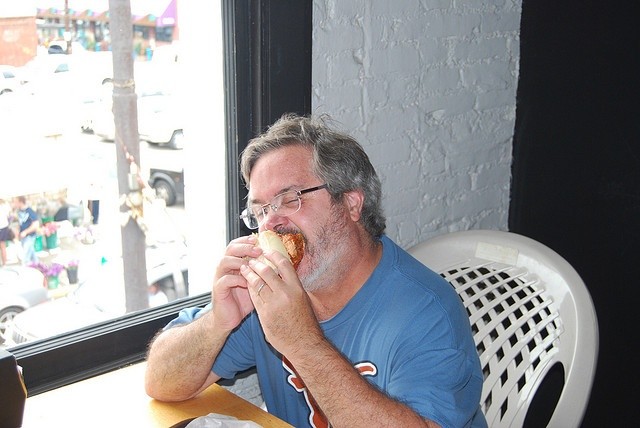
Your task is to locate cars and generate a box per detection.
[7,247,190,349]
[138,106,183,151]
[0,263,46,345]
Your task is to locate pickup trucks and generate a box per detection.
[148,164,184,206]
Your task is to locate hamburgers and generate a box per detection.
[242,231,304,280]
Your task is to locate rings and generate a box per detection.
[256,283,267,297]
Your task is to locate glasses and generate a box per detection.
[240,186,325,231]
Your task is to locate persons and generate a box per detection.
[0,197,10,268]
[144,112,489,428]
[14,193,40,268]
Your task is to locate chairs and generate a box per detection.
[406,230,599,428]
